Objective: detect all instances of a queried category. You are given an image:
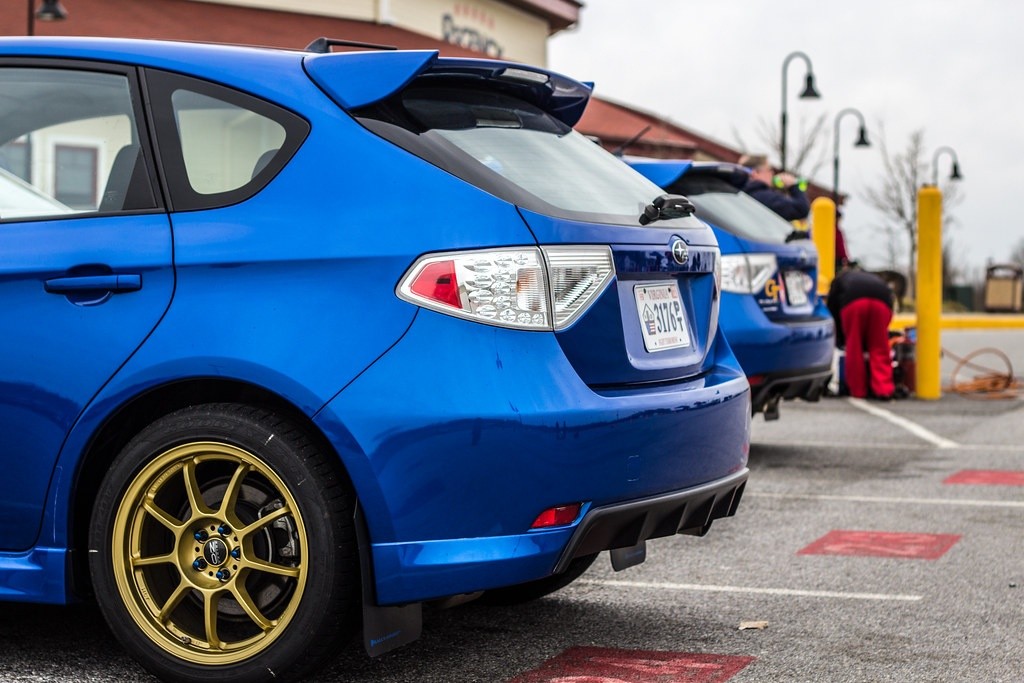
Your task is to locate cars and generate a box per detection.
[1,38,838,683]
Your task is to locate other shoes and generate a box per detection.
[881,385,908,401]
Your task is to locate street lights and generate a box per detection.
[831,107,872,194]
[778,51,823,172]
[930,145,963,189]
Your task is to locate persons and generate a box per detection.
[827,271,895,401]
